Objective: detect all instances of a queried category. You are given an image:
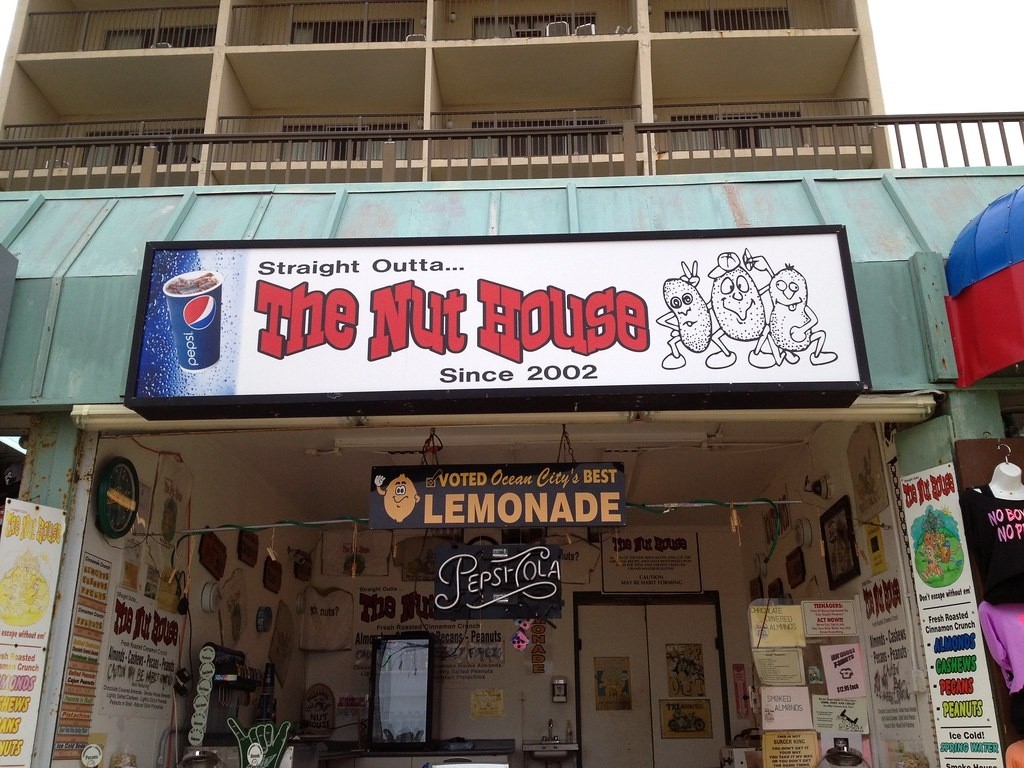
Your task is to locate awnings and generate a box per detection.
[944,184,1024,386]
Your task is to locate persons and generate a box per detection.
[960,463,1024,768]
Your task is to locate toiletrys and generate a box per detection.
[564,721,573,743]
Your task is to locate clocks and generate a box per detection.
[96,456,139,540]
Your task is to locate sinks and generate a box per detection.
[523,741,578,758]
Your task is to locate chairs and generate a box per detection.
[406,34,425,41]
[575,23,596,35]
[45,160,70,168]
[546,21,570,36]
[509,25,516,38]
[150,42,171,48]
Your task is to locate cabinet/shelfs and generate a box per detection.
[185,731,515,768]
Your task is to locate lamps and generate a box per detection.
[448,11,455,23]
[417,118,423,130]
[420,16,426,29]
[653,112,658,121]
[447,119,453,129]
[335,421,709,450]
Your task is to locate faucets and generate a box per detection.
[547,719,556,739]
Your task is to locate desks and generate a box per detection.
[515,29,541,37]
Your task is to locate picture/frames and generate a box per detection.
[819,494,861,591]
